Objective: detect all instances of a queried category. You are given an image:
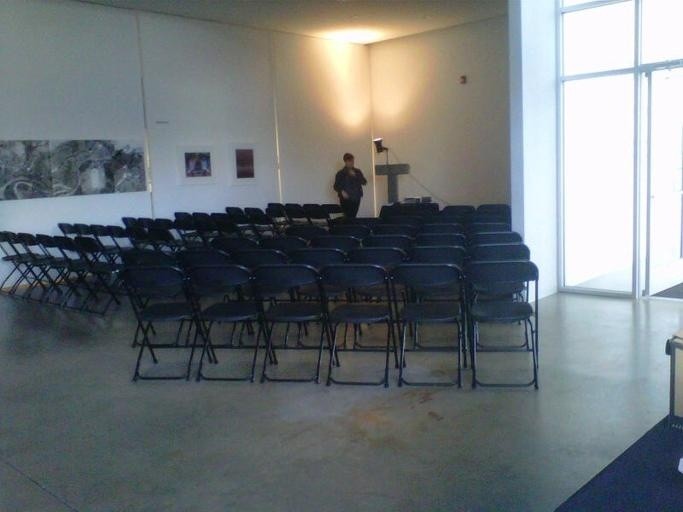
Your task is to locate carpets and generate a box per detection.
[552,412,682,511]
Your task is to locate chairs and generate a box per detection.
[120,199,540,392]
[1,223,120,316]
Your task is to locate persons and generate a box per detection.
[333,153,367,219]
